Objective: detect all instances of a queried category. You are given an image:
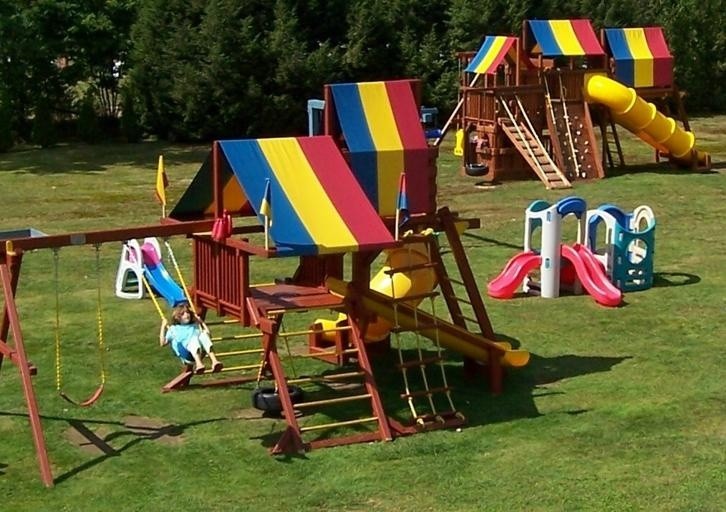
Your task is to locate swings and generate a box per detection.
[123,241,206,365]
[54,248,105,406]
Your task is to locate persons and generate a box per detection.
[158,302,224,373]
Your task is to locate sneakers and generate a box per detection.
[194,364,206,375]
[212,361,224,373]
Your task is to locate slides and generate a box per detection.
[325,276,530,367]
[313,243,438,343]
[583,72,711,173]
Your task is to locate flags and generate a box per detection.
[397,170,411,231]
[258,177,273,227]
[151,154,170,208]
